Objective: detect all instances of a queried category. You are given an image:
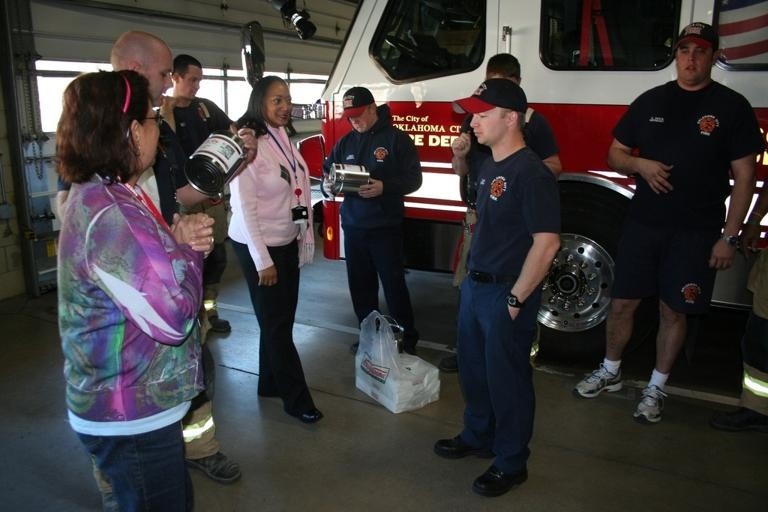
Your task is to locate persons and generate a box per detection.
[320,87,424,356]
[58,28,259,482]
[433,77,562,496]
[705,176,768,434]
[154,54,235,334]
[571,21,763,424]
[226,76,323,423]
[438,54,561,372]
[56,70,216,511]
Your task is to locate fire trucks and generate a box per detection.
[239,1,768,370]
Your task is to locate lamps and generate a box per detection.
[271,0,316,40]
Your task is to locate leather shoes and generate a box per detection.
[297,410,322,423]
[434,435,495,459]
[473,466,527,496]
[712,405,768,431]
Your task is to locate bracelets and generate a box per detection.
[747,211,762,225]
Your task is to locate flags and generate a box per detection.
[716,0,767,65]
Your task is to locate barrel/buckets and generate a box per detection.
[183,131,249,198]
[328,162,371,196]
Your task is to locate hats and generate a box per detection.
[341,87,375,118]
[452,78,527,114]
[674,22,719,50]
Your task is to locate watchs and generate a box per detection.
[723,233,738,247]
[507,292,523,308]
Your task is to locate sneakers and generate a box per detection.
[103,492,119,511]
[210,317,231,333]
[633,385,667,423]
[439,357,458,373]
[186,452,241,484]
[574,363,623,399]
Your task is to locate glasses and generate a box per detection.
[148,114,162,124]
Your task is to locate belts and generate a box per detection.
[470,271,494,282]
